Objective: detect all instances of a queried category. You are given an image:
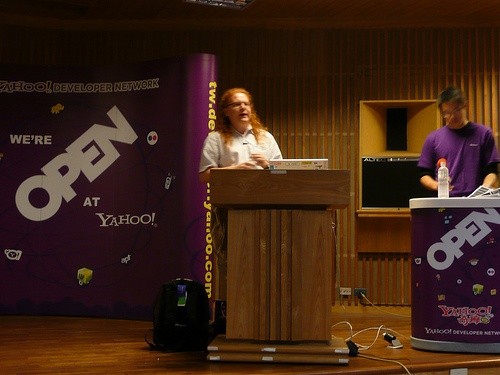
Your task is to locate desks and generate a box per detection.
[409,197,500,354]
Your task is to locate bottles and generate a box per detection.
[438,162,449,198]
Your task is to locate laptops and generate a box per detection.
[269,159,329,170]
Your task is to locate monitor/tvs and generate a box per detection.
[361,157,432,210]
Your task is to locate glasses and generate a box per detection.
[443,105,466,118]
[224,102,250,107]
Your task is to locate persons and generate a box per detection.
[418,88,500,197]
[199,88,283,301]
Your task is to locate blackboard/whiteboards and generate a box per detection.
[359,154,439,211]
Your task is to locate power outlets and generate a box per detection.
[340,288,351,295]
[354,288,367,297]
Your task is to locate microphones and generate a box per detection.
[243,142,248,144]
[437,159,446,167]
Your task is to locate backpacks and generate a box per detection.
[153,278,210,351]
